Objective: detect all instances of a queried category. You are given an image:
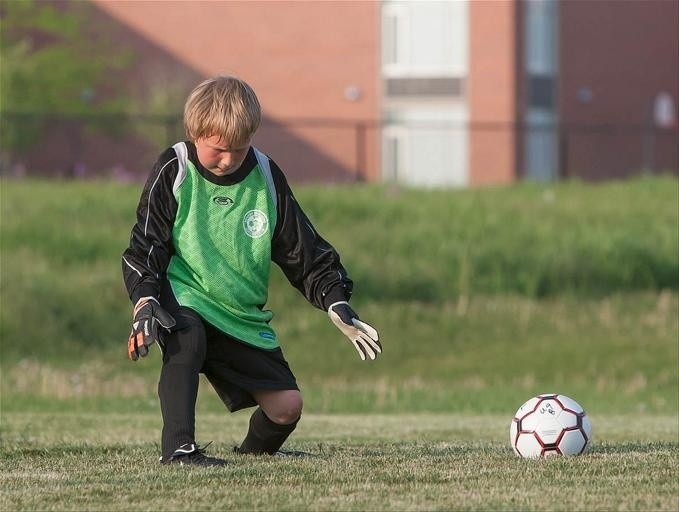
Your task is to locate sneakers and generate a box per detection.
[159,443,228,469]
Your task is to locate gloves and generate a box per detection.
[128,296,177,361]
[327,301,382,360]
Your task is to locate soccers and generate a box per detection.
[510,391,591,458]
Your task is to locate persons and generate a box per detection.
[118,75,384,470]
